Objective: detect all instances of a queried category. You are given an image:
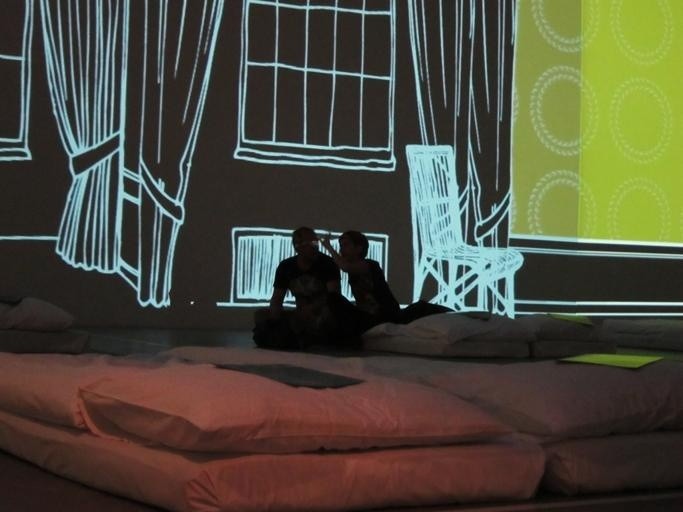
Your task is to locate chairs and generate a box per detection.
[406,144,524,319]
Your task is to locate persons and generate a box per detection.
[318,230,400,332]
[254,225,341,310]
